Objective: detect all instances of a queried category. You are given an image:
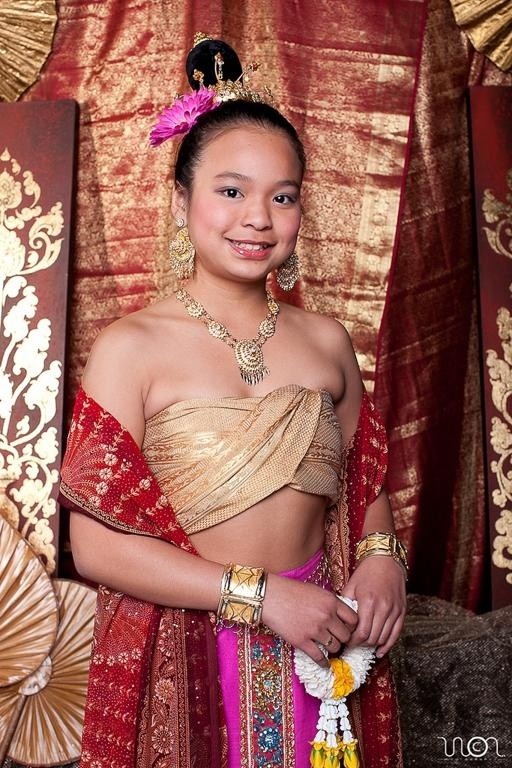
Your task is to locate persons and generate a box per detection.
[55,39,408,765]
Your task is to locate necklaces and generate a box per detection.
[174,273,281,386]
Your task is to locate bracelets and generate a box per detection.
[216,562,267,629]
[353,530,409,570]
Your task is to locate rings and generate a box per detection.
[323,634,335,648]
